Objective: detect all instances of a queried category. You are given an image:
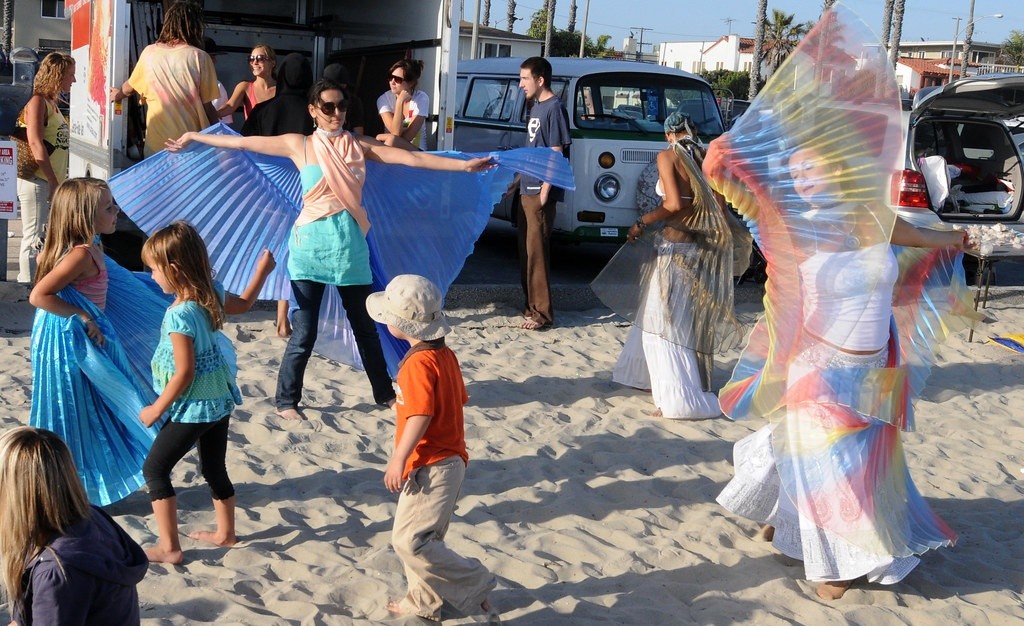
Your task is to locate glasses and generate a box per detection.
[388,74,407,84]
[317,99,348,115]
[247,54,269,62]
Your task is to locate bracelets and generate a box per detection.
[636,214,647,233]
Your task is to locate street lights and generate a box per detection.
[950,13,1003,83]
[495,17,523,29]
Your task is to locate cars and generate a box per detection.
[578,100,755,128]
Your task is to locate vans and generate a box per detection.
[455,57,727,269]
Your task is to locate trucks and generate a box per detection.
[60,0,462,271]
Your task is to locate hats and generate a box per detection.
[365,273,452,342]
[664,112,695,134]
[200,37,229,55]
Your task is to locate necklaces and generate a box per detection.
[827,215,862,250]
[316,127,343,137]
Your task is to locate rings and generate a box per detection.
[626,234,629,238]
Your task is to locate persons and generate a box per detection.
[626,110,736,420]
[109,2,430,338]
[0,426,150,626]
[162,78,501,422]
[16,51,77,283]
[501,56,573,330]
[364,272,497,623]
[28,177,278,565]
[700,132,977,602]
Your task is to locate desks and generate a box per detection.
[943,222,1024,343]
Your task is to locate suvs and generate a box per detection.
[784,72,1024,232]
